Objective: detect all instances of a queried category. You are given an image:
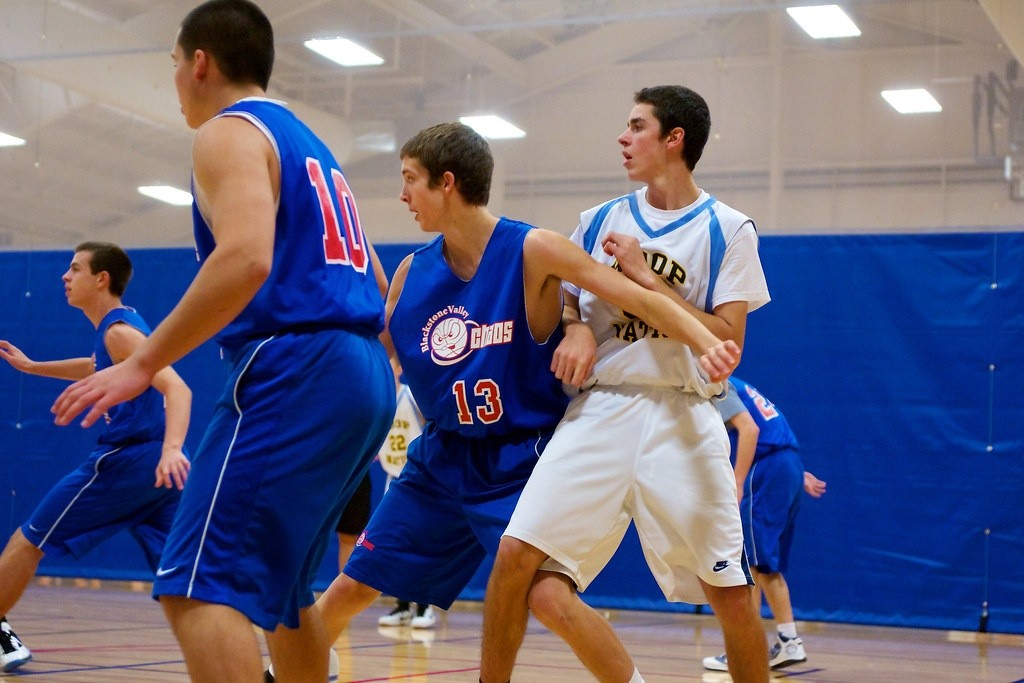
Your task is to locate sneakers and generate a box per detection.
[378,599,413,625]
[264,648,338,681]
[411,605,435,628]
[702,652,729,671]
[768,632,806,669]
[0,616,32,671]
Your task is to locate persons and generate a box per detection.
[378,356,437,628]
[263,122,741,682]
[0,243,192,670]
[477,84,770,683]
[336,471,371,574]
[702,378,825,672]
[49,0,387,683]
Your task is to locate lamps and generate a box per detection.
[785,4,862,38]
[881,87,943,116]
[303,33,384,67]
[460,114,527,139]
[138,184,194,205]
